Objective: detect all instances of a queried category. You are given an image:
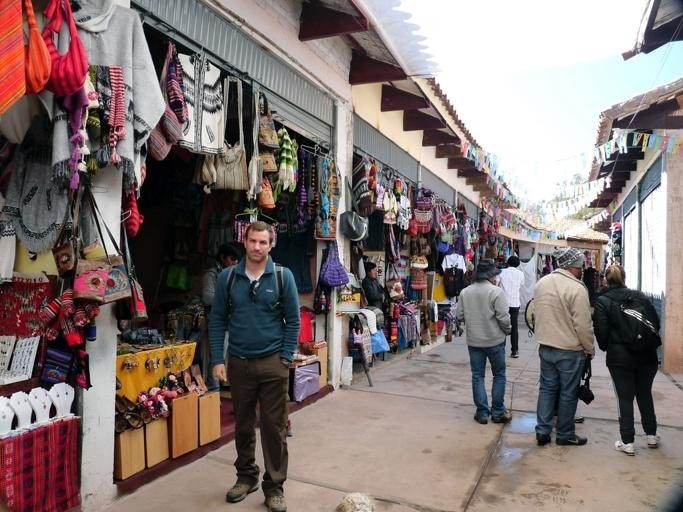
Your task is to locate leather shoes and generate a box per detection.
[474,412,487,424]
[492,410,512,423]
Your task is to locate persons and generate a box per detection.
[201,243,240,389]
[497,255,525,358]
[208,221,301,512]
[363,262,386,307]
[534,246,596,447]
[593,265,661,460]
[456,259,512,424]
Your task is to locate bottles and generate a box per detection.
[318,290,324,298]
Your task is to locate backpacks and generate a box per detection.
[606,289,662,352]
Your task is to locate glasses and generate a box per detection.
[249,280,258,305]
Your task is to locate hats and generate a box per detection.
[470,257,502,281]
[83,74,100,111]
[551,245,585,269]
[59,88,89,112]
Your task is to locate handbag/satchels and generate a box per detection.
[372,321,388,354]
[339,211,369,242]
[148,42,188,163]
[313,239,349,315]
[53,175,149,323]
[25,1,52,95]
[211,76,281,209]
[40,2,89,97]
[385,187,456,301]
[352,314,364,345]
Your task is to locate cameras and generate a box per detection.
[578,384,594,404]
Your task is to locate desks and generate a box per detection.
[115,342,196,398]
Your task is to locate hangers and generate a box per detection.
[195,38,207,61]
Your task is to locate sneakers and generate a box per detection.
[645,431,660,448]
[225,477,260,502]
[510,351,519,358]
[536,415,587,445]
[264,496,288,512]
[614,441,634,456]
